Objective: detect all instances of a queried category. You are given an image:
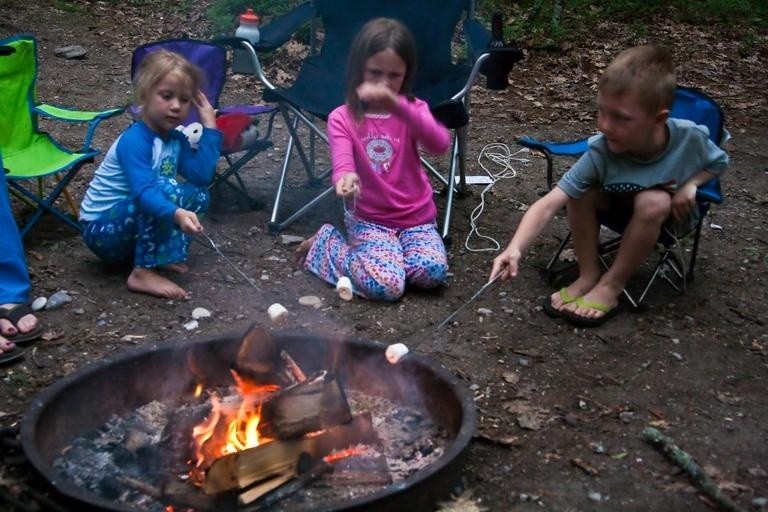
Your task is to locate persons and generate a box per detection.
[487,43,731,327]
[293,16,454,304]
[78,47,225,300]
[0,151,46,365]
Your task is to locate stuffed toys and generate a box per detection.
[174,111,261,153]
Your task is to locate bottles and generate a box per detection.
[232,8,261,76]
[486,13,509,90]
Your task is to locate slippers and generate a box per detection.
[0,302,44,363]
[544,287,623,328]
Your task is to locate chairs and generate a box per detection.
[261,0,516,242]
[128,38,281,211]
[515,85,728,310]
[0,38,124,240]
